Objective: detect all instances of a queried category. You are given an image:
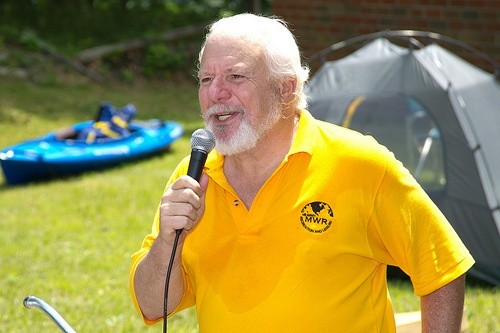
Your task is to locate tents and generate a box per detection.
[301,30,500,289]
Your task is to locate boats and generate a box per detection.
[0,104,184,185]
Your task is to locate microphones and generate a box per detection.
[174,129,216,232]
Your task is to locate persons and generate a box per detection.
[128,12,476,333]
